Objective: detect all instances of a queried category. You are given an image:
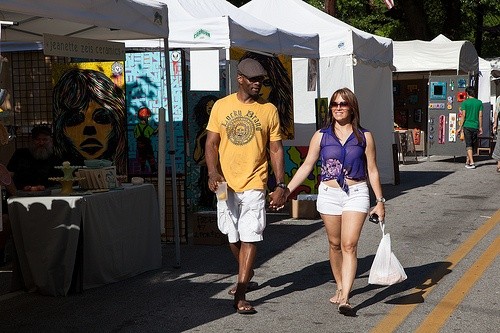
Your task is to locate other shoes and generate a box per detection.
[11,273,25,291]
[496,166,500,173]
[465,164,476,169]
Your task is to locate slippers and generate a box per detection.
[233,298,253,313]
[228,281,258,295]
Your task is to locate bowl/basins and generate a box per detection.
[84,160,101,167]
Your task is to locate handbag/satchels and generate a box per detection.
[367,222,408,285]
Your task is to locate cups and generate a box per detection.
[216,182,228,202]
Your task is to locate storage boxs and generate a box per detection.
[291,198,319,219]
[194,211,228,245]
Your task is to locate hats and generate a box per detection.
[32,126,52,140]
[237,58,268,79]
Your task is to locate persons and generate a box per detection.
[0,126,64,266]
[455,86,484,169]
[492,95,500,172]
[204,58,287,314]
[268,88,386,314]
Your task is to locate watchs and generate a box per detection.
[275,182,286,189]
[375,198,386,203]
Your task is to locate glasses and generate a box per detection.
[329,101,351,109]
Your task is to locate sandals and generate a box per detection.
[329,289,342,305]
[338,301,354,316]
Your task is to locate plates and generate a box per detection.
[83,166,104,168]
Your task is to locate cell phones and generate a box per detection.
[369,213,379,224]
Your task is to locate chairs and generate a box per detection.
[476,135,493,156]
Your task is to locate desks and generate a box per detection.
[7,183,161,296]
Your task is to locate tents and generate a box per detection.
[0,0,500,267]
